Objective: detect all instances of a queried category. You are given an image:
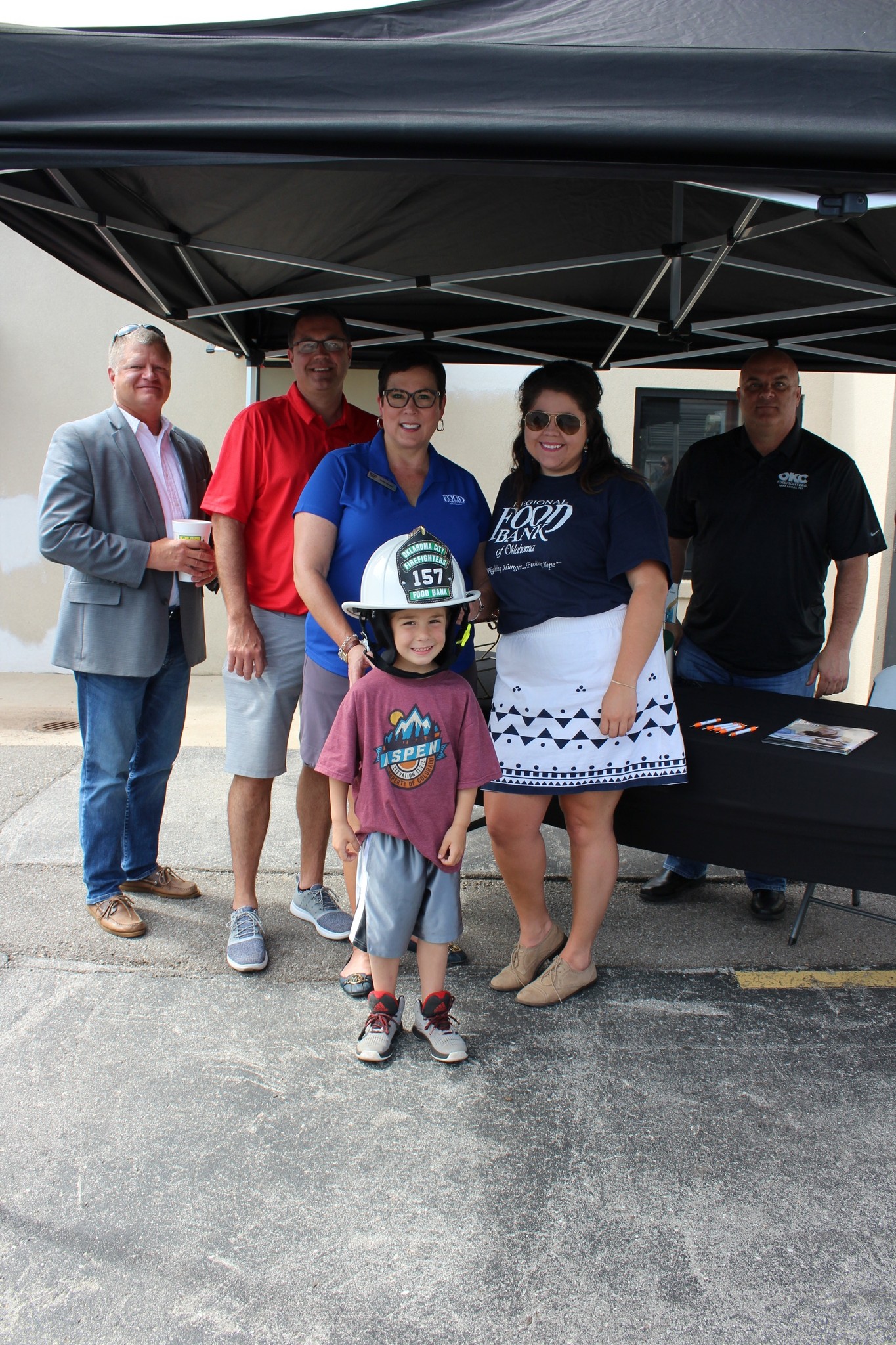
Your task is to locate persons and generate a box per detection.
[292,346,495,997]
[481,359,688,1007]
[639,349,887,923]
[312,527,504,1065]
[37,323,219,937]
[200,300,384,973]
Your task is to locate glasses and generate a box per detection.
[108,324,166,357]
[289,339,347,355]
[521,411,588,436]
[381,387,442,408]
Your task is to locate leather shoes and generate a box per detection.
[87,897,146,937]
[116,866,200,900]
[638,870,706,902]
[751,888,786,919]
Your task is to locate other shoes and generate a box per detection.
[488,925,569,991]
[517,957,596,1006]
[339,954,373,995]
[408,939,467,966]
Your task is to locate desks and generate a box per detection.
[466,650,896,896]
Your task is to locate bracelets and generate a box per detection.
[470,597,484,622]
[338,634,359,660]
[609,678,638,692]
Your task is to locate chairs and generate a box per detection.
[788,665,896,945]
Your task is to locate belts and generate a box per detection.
[168,607,180,620]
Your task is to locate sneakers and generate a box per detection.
[226,906,267,971]
[410,993,468,1062]
[289,885,353,940]
[356,991,404,1061]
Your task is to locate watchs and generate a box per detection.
[341,639,363,664]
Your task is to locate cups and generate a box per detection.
[171,518,212,583]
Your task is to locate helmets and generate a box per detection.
[342,529,481,620]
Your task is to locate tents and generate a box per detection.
[0,0,896,371]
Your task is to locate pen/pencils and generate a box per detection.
[716,725,747,734]
[702,722,738,731]
[710,723,745,731]
[728,726,758,736]
[689,718,721,727]
